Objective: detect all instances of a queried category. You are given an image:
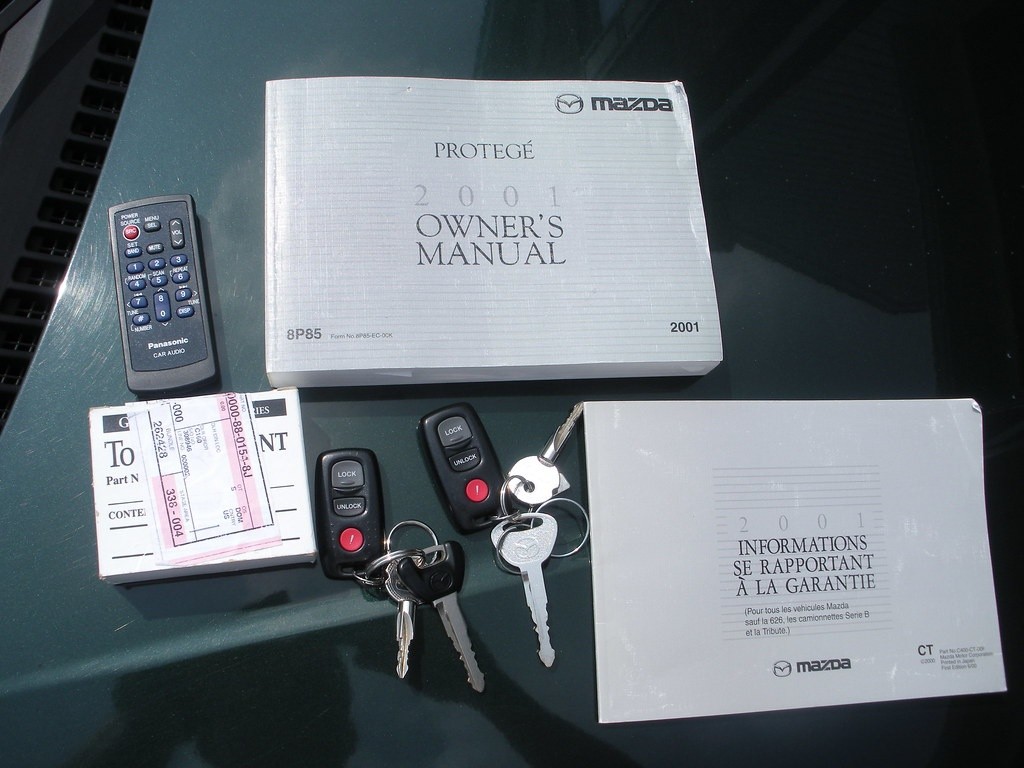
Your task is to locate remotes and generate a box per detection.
[108,193,217,394]
[418,400,518,534]
[315,447,390,581]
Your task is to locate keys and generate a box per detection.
[416,400,589,668]
[315,446,488,695]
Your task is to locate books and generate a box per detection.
[585,402,1007,725]
[89,385,314,586]
[264,76,725,391]
